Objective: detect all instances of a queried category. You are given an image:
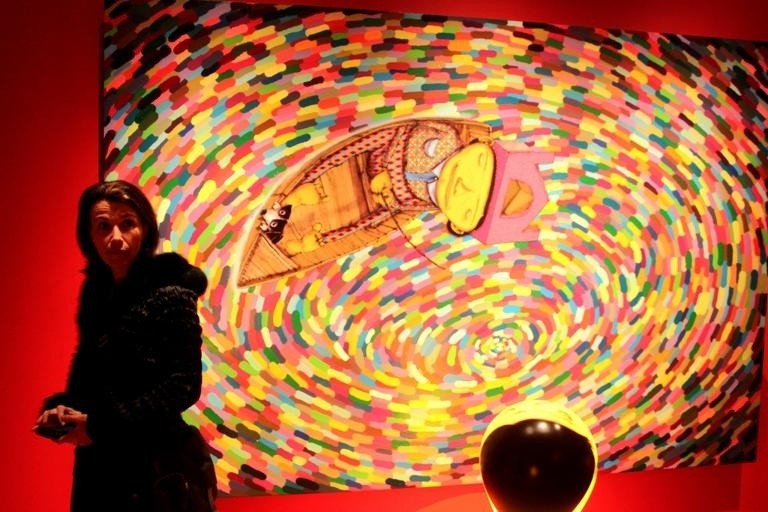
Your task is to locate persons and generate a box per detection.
[35,180,219,511]
